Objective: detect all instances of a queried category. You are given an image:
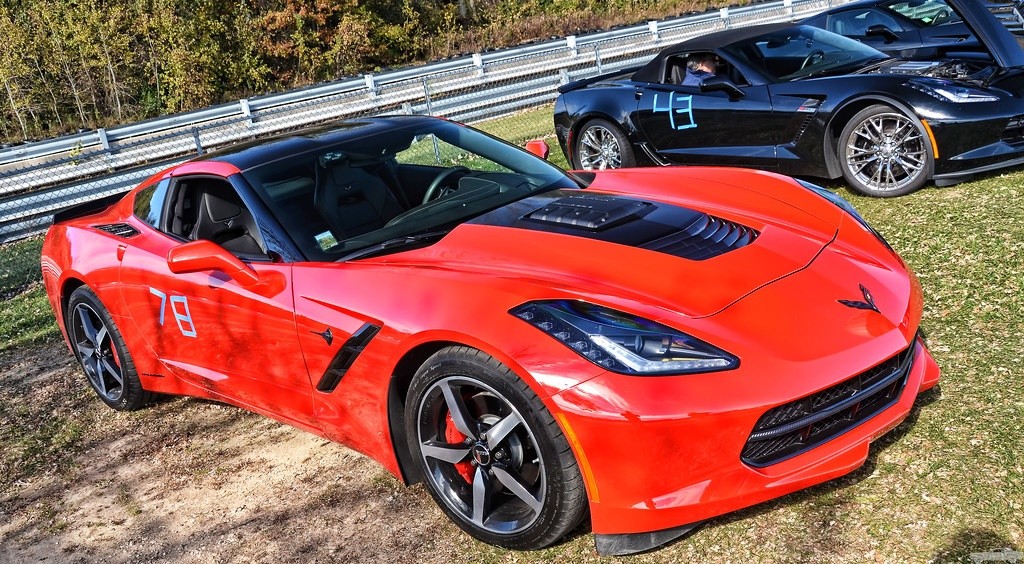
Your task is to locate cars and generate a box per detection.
[979,0,1024,34]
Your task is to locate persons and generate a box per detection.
[682,53,717,86]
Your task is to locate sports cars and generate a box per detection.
[797,0,996,67]
[552,1,1024,200]
[39,119,943,560]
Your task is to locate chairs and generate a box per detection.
[191,184,270,259]
[672,60,688,85]
[314,150,402,241]
